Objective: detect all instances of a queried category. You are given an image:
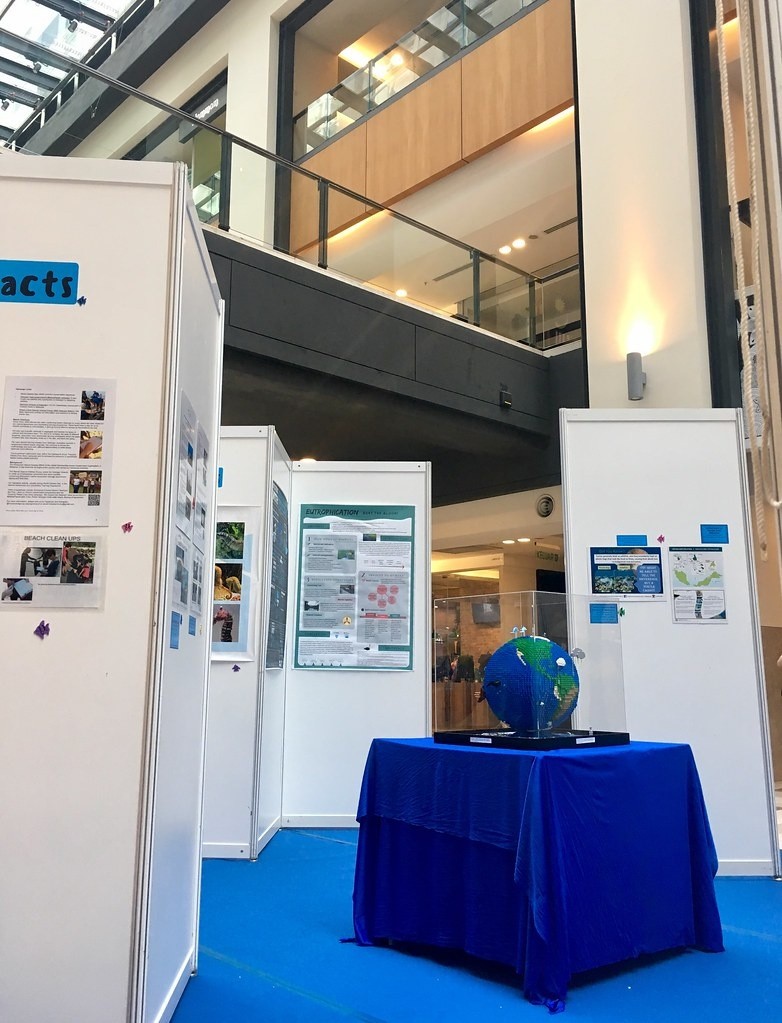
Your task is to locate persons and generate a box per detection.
[0,577,33,602]
[20,546,61,577]
[69,473,97,494]
[63,541,93,584]
[79,430,95,459]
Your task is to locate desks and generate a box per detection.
[348,739,725,1013]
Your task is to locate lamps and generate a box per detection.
[625,352,650,401]
[1,99,9,112]
[32,60,41,73]
[68,19,78,33]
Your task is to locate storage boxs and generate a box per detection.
[432,590,631,749]
